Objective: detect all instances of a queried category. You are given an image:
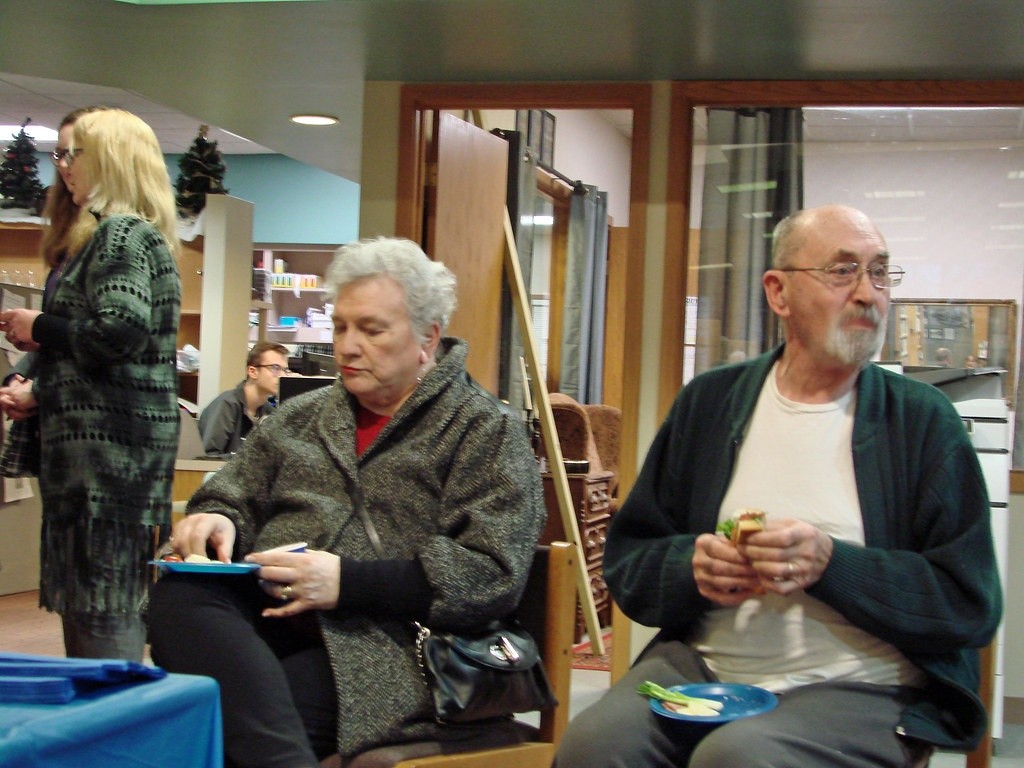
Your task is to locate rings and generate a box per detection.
[788,560,795,577]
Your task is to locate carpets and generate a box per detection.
[569,632,614,672]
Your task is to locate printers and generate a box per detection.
[874,360,1009,756]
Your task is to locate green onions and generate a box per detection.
[637,681,725,713]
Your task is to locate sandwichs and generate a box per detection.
[717,511,767,551]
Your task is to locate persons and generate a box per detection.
[145,237,546,768]
[550,203,1002,764]
[1,110,184,677]
[195,344,293,455]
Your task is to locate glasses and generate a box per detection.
[779,258,903,289]
[254,364,290,375]
[52,148,82,165]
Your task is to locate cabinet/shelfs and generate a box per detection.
[2,193,253,463]
[250,242,348,379]
[541,470,617,644]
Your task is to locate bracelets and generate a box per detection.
[282,584,292,601]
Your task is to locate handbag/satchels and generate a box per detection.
[0,417,41,479]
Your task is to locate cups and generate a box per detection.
[260,541,308,606]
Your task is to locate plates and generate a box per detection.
[147,560,259,574]
[649,681,778,723]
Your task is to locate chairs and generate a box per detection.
[528,391,624,468]
[321,540,579,768]
